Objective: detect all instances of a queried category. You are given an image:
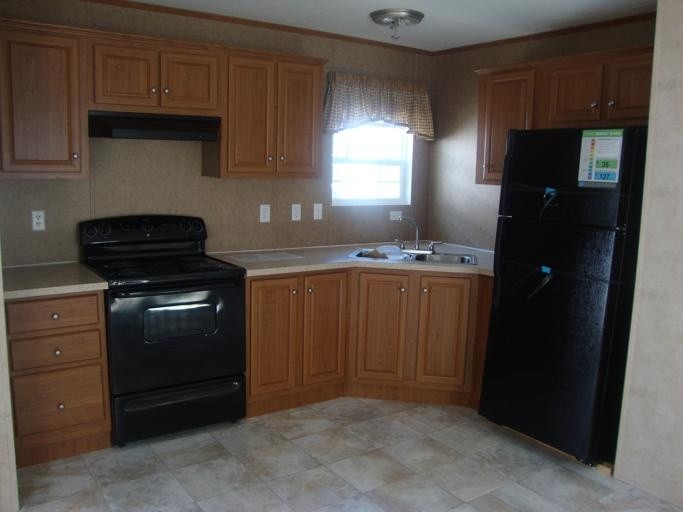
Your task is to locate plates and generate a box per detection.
[369,245,406,261]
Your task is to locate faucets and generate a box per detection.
[399,215,421,250]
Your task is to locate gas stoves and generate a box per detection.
[87,238,247,290]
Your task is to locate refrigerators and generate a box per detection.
[479,124,649,472]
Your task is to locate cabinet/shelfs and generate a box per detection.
[0,17,90,179]
[475,44,653,185]
[88,29,223,117]
[244,271,347,419]
[202,45,329,178]
[5,291,113,470]
[349,267,479,408]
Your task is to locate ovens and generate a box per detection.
[103,287,247,448]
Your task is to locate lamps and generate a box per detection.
[370,7,424,39]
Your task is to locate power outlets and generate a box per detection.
[31,210,46,232]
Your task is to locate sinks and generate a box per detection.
[413,251,480,267]
[348,245,413,264]
